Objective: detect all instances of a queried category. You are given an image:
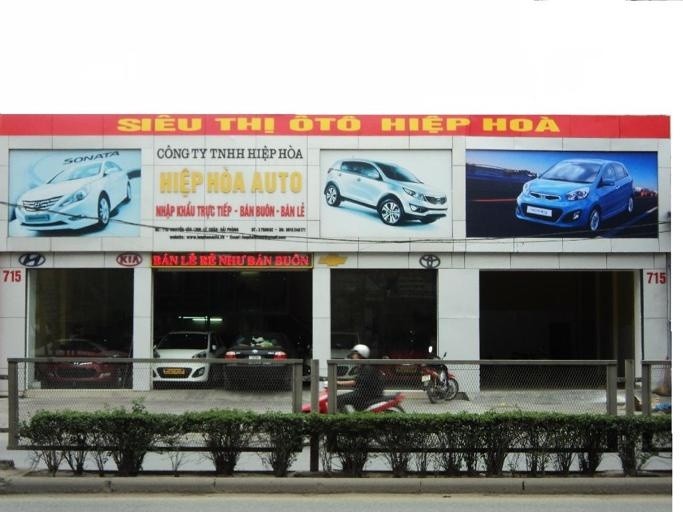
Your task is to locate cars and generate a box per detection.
[324,157,448,225]
[16,160,131,230]
[515,158,634,232]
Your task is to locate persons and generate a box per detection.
[323,345,383,415]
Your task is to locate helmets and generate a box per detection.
[353,344,371,358]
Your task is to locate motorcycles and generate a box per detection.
[302,384,407,415]
[420,346,459,404]
